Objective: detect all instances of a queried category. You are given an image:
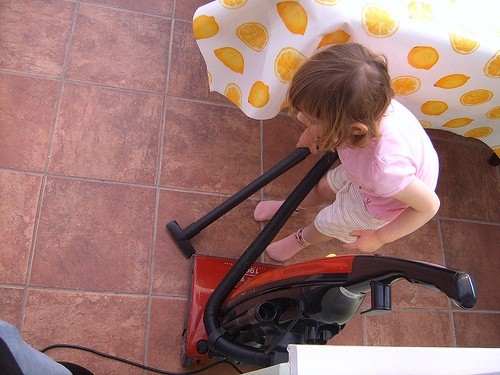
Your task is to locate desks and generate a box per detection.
[310,0,499,167]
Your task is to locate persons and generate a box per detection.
[254,43,439,264]
